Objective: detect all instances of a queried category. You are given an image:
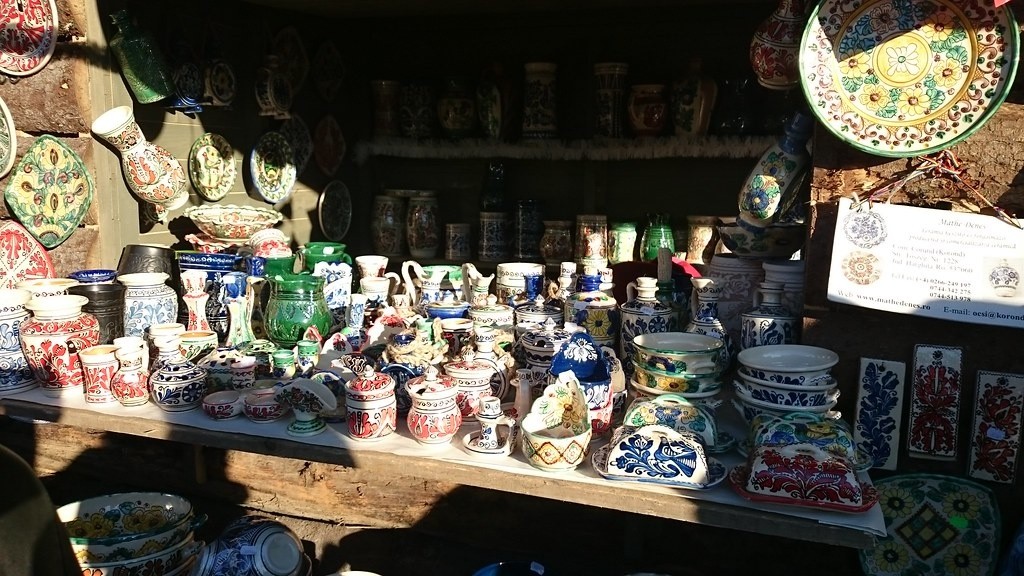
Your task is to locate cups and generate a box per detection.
[78,228,629,472]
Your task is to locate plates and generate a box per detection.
[1,1,353,289]
[798,0,1021,157]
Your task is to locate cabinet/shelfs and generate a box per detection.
[353,132,815,276]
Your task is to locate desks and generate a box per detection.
[0,388,889,576]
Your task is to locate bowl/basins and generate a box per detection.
[628,332,841,427]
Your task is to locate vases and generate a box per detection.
[90,106,189,210]
[670,49,718,137]
[749,0,808,91]
